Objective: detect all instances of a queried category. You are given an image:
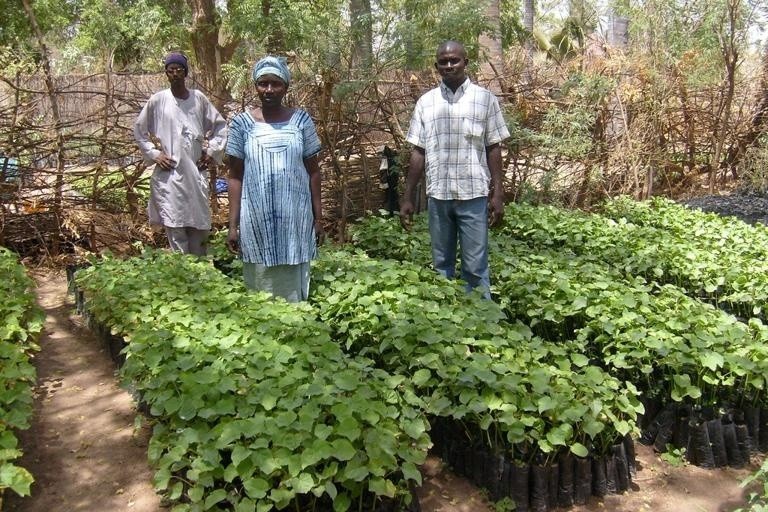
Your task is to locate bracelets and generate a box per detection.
[315,219,323,223]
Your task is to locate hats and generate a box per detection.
[164,53,189,74]
[251,56,289,82]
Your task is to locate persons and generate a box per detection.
[398,40,512,300]
[223,56,326,304]
[134,53,228,257]
